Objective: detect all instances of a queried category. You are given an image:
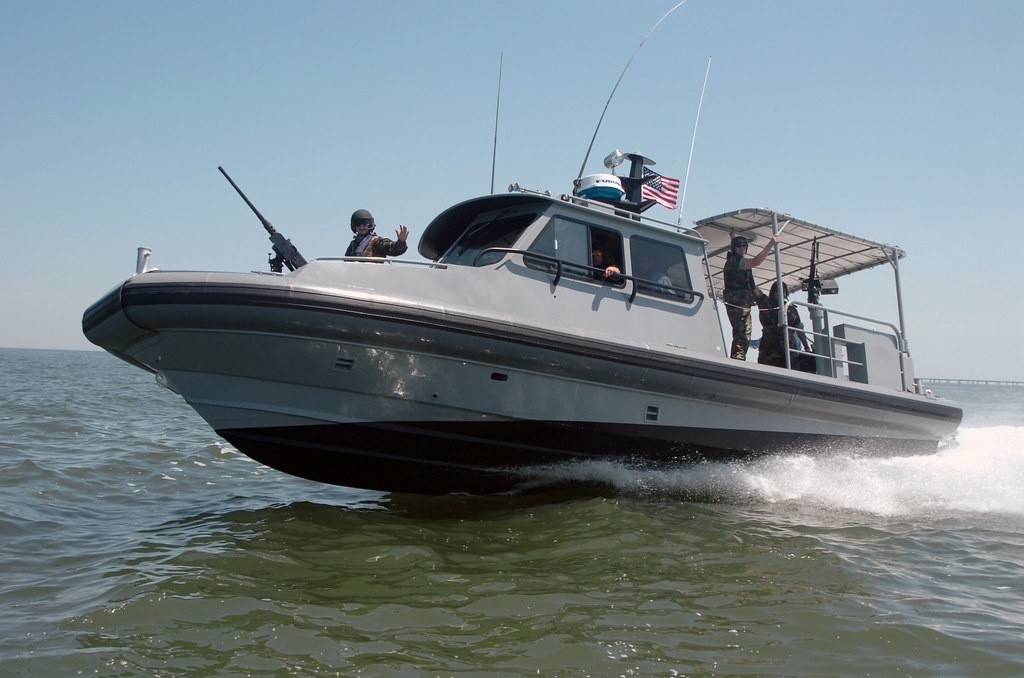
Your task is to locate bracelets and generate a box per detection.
[805,347,810,349]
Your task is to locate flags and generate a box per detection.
[642,166,680,209]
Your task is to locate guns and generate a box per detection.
[806,236,824,312]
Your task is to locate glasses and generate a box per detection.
[592,253,603,257]
[736,245,747,248]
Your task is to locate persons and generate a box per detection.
[591,248,622,284]
[723,237,811,369]
[344,209,409,264]
[642,258,676,295]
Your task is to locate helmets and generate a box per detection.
[350,209,376,233]
[770,281,788,298]
[731,236,748,254]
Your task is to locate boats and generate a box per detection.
[83,0,963,494]
